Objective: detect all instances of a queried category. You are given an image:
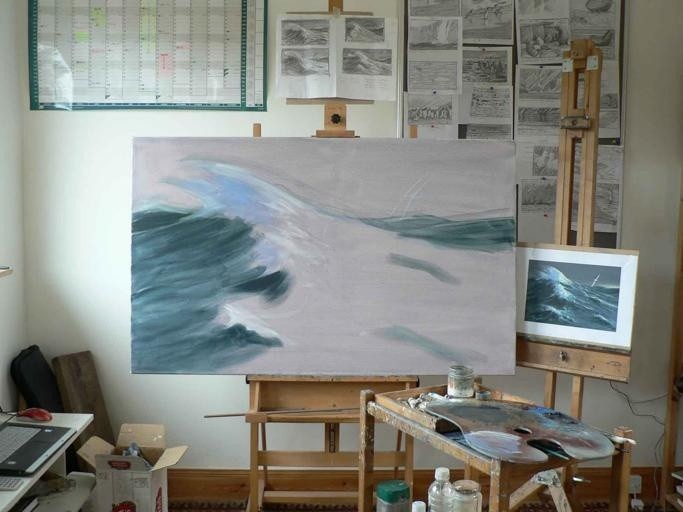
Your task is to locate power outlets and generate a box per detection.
[630,475,642,493]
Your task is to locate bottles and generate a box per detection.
[412,501,426,512]
[428,467,453,512]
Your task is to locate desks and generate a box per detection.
[0,412,96,512]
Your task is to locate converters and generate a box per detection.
[631,499,644,508]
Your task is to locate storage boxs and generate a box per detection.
[76,422,189,512]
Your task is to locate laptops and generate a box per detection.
[0,415,77,476]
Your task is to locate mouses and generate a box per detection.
[16,407,51,422]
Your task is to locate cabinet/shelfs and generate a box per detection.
[245,373,419,512]
[358,377,633,512]
